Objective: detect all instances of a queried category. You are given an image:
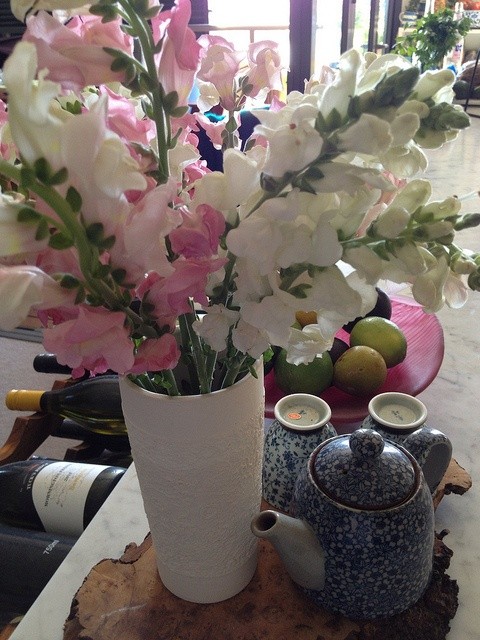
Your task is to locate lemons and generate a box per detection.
[349,317,408,368]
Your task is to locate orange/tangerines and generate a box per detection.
[273,348,333,397]
[333,346,388,398]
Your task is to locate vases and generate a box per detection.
[117,353,266,604]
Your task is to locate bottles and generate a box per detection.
[5,374,126,435]
[1,525,79,607]
[1,461,125,536]
[32,352,112,375]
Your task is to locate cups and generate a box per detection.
[263,396,339,512]
[360,393,427,449]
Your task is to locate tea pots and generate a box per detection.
[251,427,452,619]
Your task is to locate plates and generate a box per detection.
[263,300,444,422]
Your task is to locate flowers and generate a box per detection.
[0,0,480,398]
